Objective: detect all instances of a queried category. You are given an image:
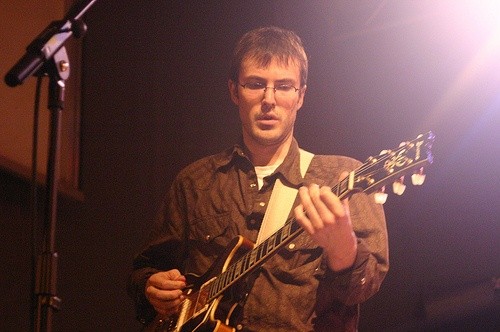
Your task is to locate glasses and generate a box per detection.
[237,80,300,97]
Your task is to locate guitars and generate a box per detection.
[142,131,437,332]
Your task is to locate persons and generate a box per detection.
[122,25,392,332]
